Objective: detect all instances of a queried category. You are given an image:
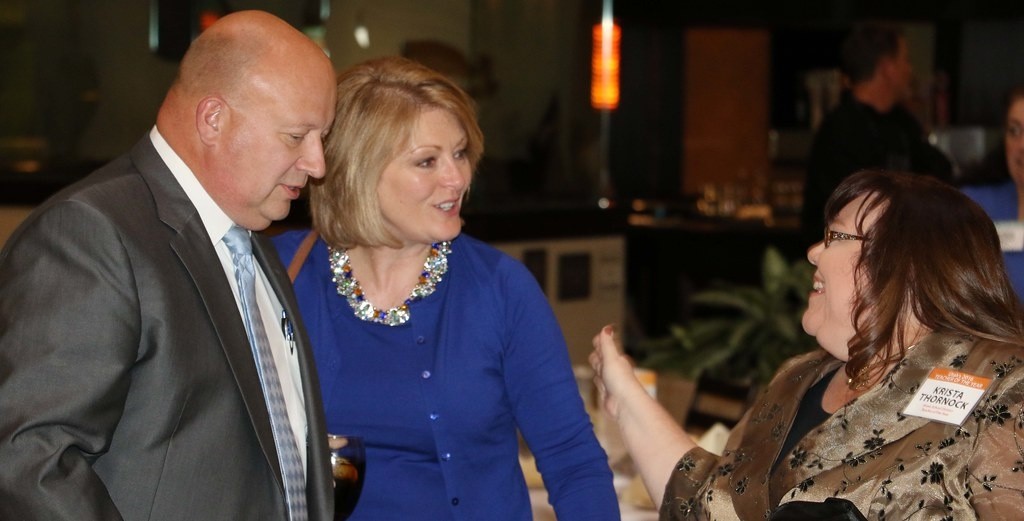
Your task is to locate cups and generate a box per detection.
[326,436,366,521]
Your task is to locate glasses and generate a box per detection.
[825,227,874,249]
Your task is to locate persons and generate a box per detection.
[0,10,1024,521]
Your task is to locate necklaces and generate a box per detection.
[849,341,921,391]
[329,239,452,326]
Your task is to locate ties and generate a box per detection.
[222,224,309,521]
